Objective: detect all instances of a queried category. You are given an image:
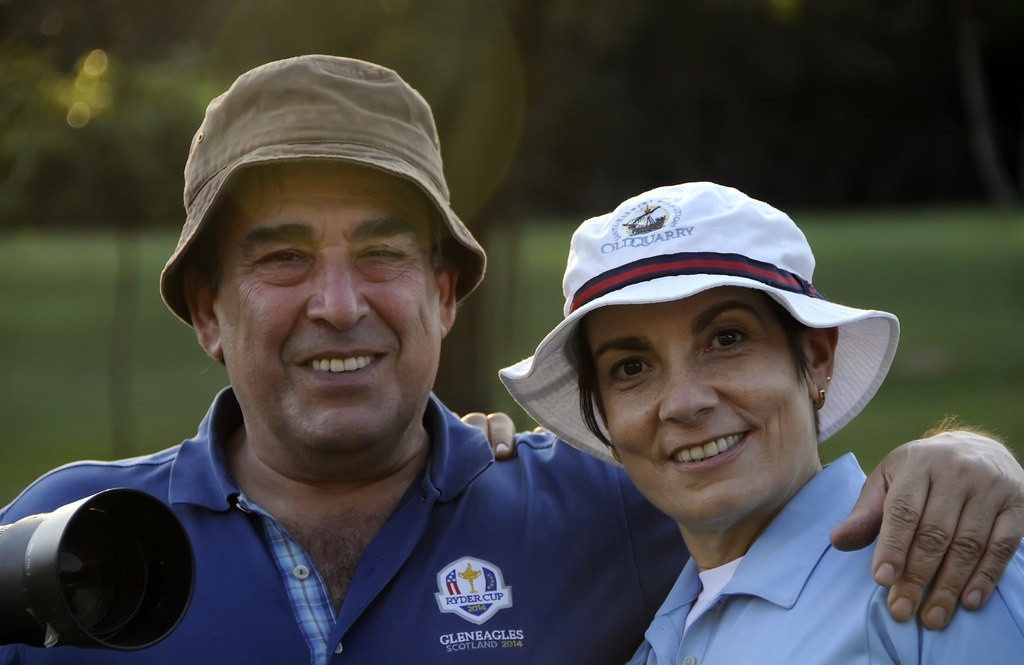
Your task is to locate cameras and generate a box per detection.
[0,487,196,651]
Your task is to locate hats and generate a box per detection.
[498,182,900,470]
[159,53,488,330]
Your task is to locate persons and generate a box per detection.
[458,180,1024,665]
[1,54,1023,665]
